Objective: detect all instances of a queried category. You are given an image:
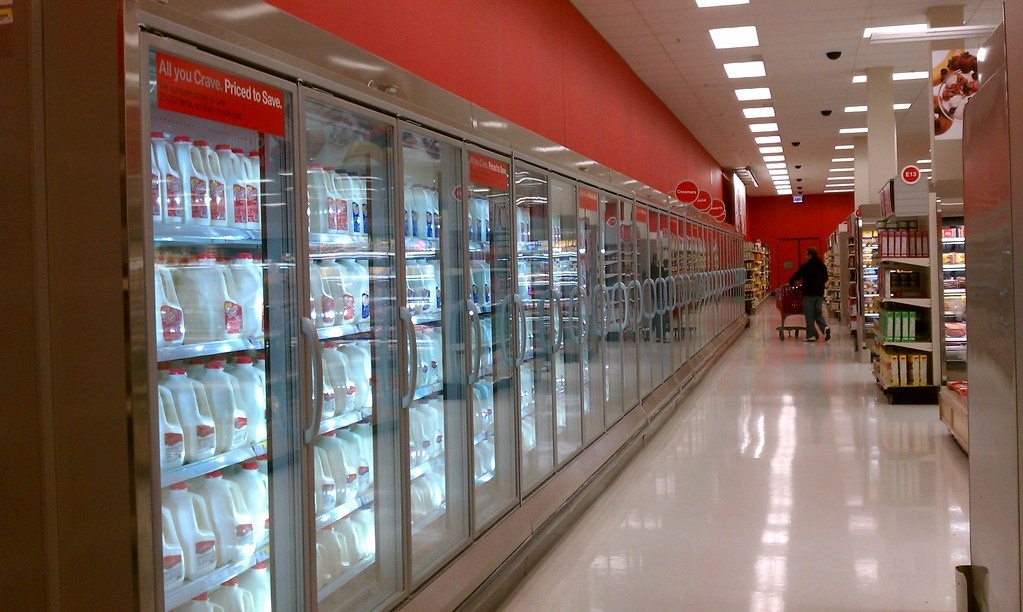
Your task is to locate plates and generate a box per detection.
[938,69,979,125]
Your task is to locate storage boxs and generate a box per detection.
[875,230,928,386]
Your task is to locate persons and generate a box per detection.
[789,247,831,342]
[650,254,669,343]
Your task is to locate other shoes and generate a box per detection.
[824,327,831,341]
[803,336,816,342]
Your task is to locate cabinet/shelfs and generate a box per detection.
[820,210,879,365]
[744,246,770,314]
[868,193,967,405]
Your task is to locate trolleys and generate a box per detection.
[776,284,807,341]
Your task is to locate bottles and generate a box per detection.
[146,129,591,612]
